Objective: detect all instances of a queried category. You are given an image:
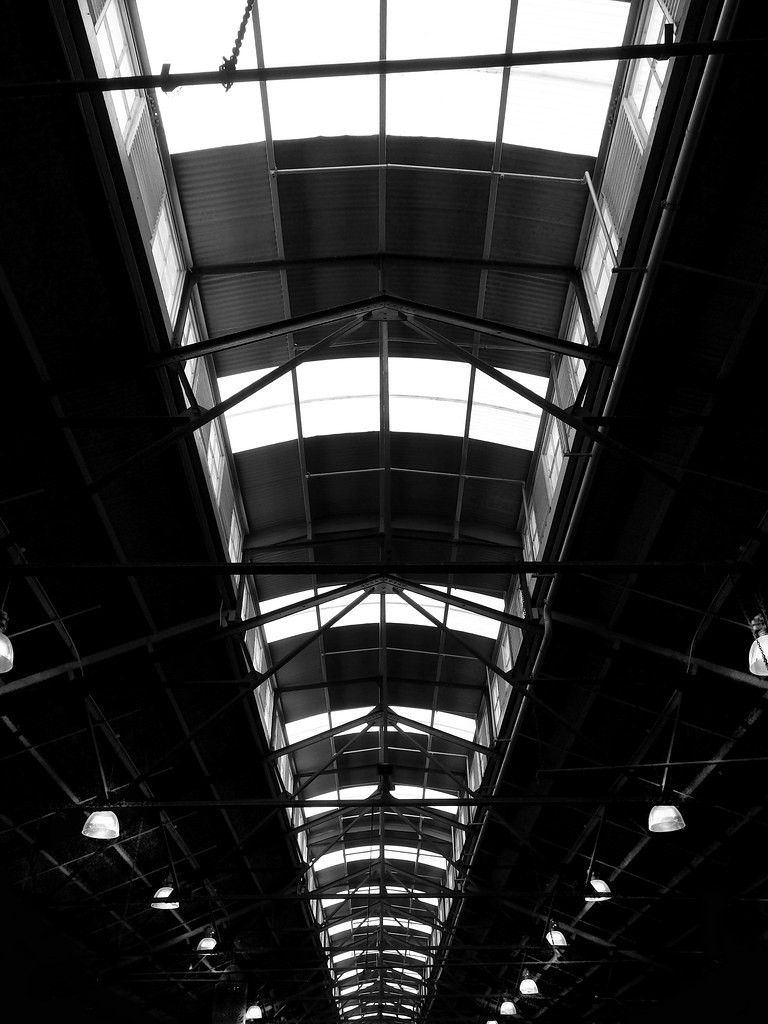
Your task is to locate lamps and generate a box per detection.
[244,979,263,1019]
[196,895,223,955]
[486,1011,498,1024]
[519,965,538,994]
[586,839,613,902]
[500,980,516,1015]
[0,577,14,674]
[546,877,567,945]
[648,706,687,833]
[151,821,180,910]
[81,713,120,840]
[742,564,768,676]
